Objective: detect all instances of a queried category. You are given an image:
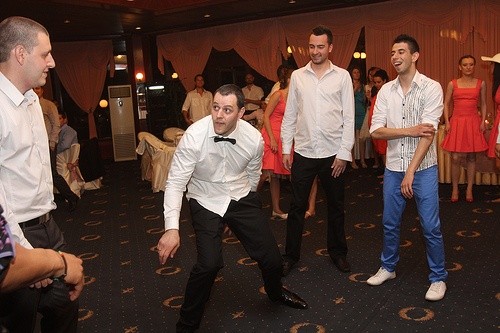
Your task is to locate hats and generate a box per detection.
[480,53,500,64]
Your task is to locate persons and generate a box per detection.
[182,75,213,127]
[239,64,298,220]
[0,15,81,333]
[35,86,79,210]
[0,212,86,301]
[156,82,309,333]
[367,33,450,303]
[279,27,355,276]
[482,49,500,204]
[443,53,487,203]
[341,65,389,187]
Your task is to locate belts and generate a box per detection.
[19,212,52,227]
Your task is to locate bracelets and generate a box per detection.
[52,248,67,282]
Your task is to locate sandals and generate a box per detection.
[304,212,317,220]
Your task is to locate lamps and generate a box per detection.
[136,73,143,83]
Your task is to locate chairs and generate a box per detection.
[53,143,85,200]
[136,127,184,192]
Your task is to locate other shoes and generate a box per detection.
[69,195,80,211]
[282,261,300,276]
[378,181,383,184]
[378,175,383,178]
[372,164,381,168]
[361,163,367,167]
[351,164,358,170]
[334,257,352,271]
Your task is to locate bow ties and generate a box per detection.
[214,137,236,145]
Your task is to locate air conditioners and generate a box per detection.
[108,85,137,161]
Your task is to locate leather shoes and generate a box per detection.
[269,287,308,309]
[176,322,193,333]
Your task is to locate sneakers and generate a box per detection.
[425,281,447,301]
[367,268,396,285]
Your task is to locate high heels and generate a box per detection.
[262,205,271,208]
[272,211,288,220]
[466,192,474,202]
[452,191,461,202]
